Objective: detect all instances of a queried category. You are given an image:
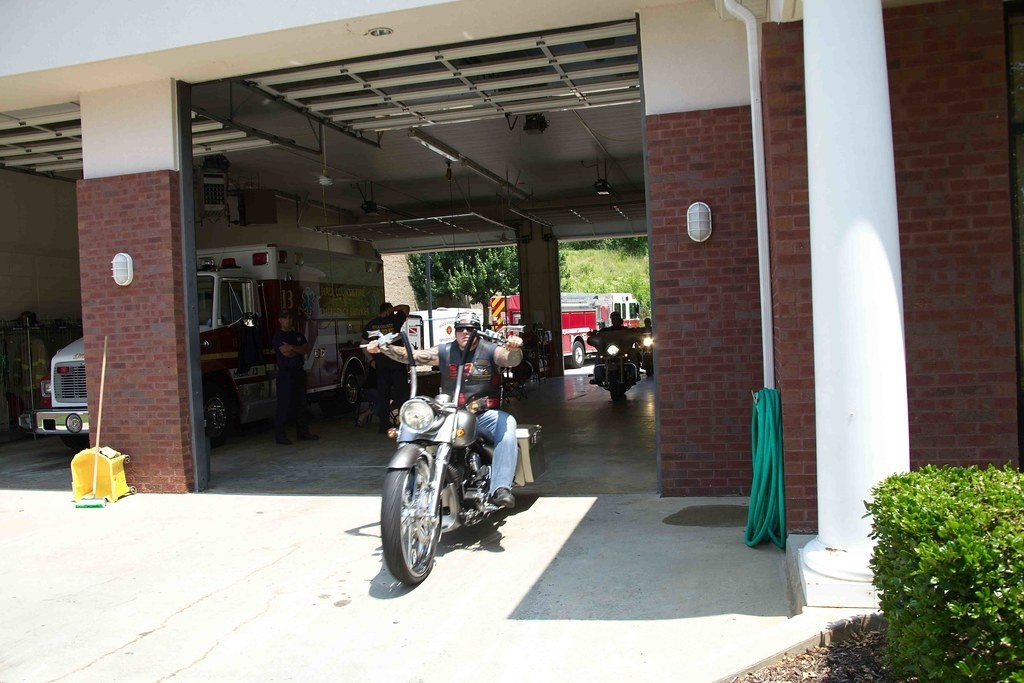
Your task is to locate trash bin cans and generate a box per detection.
[416,371,442,397]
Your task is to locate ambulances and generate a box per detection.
[399,307,484,372]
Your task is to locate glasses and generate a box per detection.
[456,327,473,332]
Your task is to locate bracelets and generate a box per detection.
[369,359,374,364]
[288,345,294,352]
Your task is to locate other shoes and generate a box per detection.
[297,432,319,440]
[276,438,293,445]
[488,487,515,508]
[379,408,391,434]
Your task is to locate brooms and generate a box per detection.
[75,336,108,508]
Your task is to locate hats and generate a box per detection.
[279,309,293,317]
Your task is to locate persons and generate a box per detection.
[360,304,410,434]
[586,311,653,387]
[367,312,524,508]
[272,309,319,446]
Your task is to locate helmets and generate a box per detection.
[610,312,621,319]
[454,312,481,331]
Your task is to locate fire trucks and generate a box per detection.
[19,243,389,449]
[487,292,640,369]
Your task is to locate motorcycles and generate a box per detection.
[358,326,546,586]
[586,329,653,402]
